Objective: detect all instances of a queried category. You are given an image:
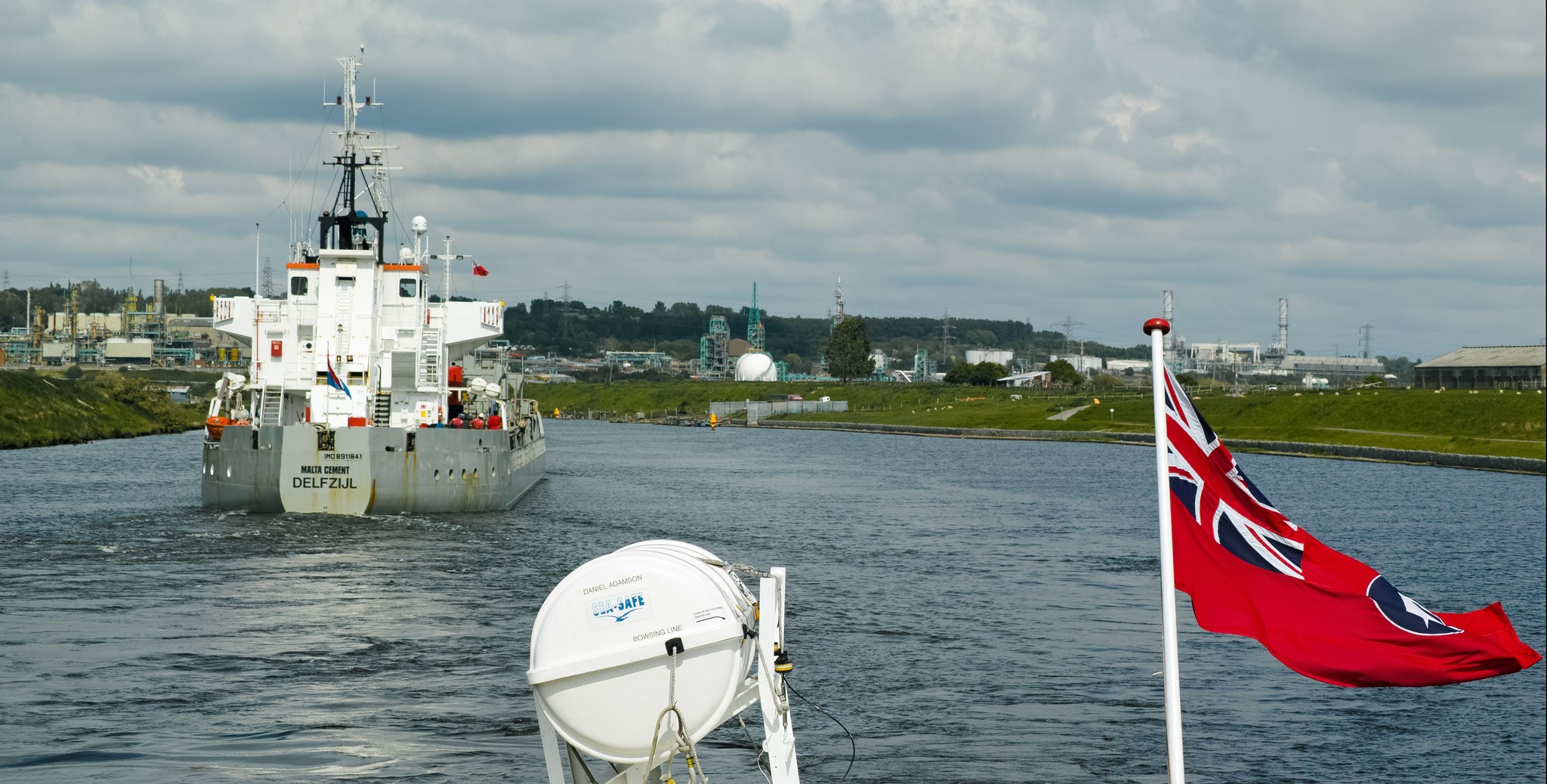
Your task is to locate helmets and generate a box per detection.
[478,413,484,418]
[458,413,464,419]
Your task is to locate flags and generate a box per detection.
[1164,363,1542,689]
[327,358,351,400]
[472,260,489,276]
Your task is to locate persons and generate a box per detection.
[450,413,464,429]
[469,413,485,429]
[487,410,503,430]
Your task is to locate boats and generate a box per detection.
[201,40,552,516]
